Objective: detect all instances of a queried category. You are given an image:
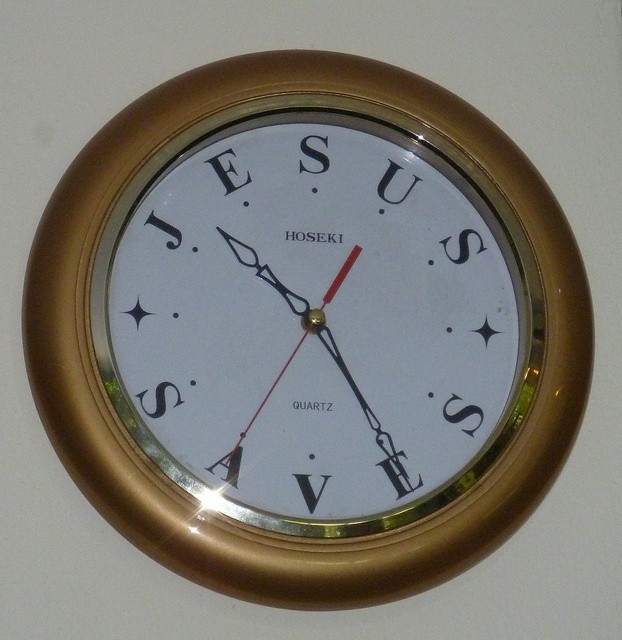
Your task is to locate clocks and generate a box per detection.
[21,49,594,612]
